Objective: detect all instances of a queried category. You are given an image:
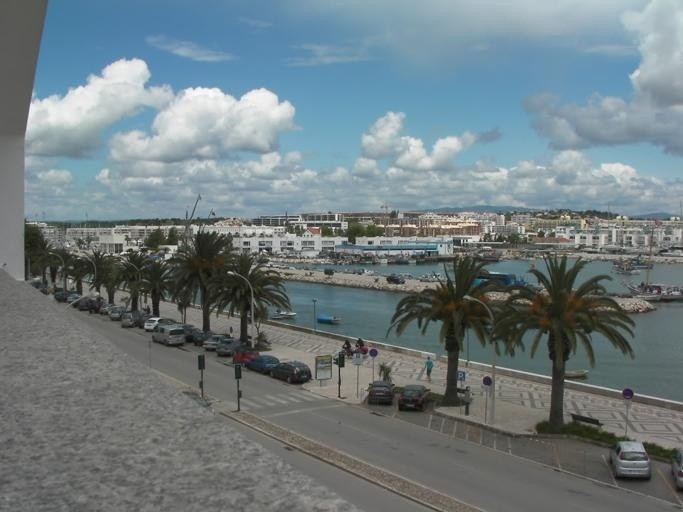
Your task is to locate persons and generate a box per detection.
[425,356,433,381]
[343,337,364,356]
[464,386,471,415]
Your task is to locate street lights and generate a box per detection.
[227,271,255,349]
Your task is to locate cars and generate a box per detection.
[398,385,433,413]
[32,278,139,328]
[139,315,312,385]
[609,440,650,481]
[670,450,683,488]
[367,380,395,406]
[268,263,291,269]
[387,272,406,284]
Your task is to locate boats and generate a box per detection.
[472,246,503,262]
[271,309,296,318]
[627,281,683,301]
[611,260,654,276]
[318,314,342,322]
[417,249,459,264]
